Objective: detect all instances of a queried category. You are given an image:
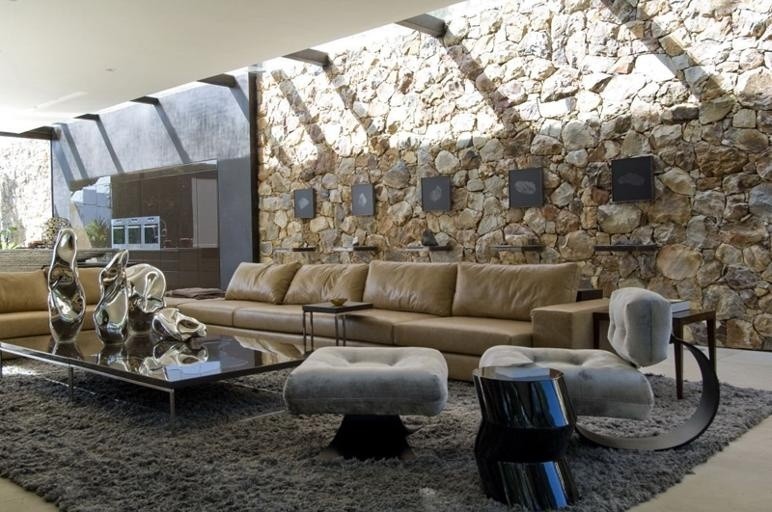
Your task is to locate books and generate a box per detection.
[667,298,691,313]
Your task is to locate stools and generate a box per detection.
[282,347,448,463]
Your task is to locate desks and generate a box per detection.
[302,302,374,346]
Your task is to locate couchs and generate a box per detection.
[177,261,609,383]
[0,267,104,359]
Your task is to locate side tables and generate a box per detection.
[593,308,716,399]
[471,365,579,508]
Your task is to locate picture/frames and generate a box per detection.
[294,188,315,219]
[612,156,655,202]
[509,167,543,208]
[421,176,451,211]
[351,183,375,216]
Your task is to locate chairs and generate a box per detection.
[479,288,719,452]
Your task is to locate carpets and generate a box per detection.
[0,366,771,512]
